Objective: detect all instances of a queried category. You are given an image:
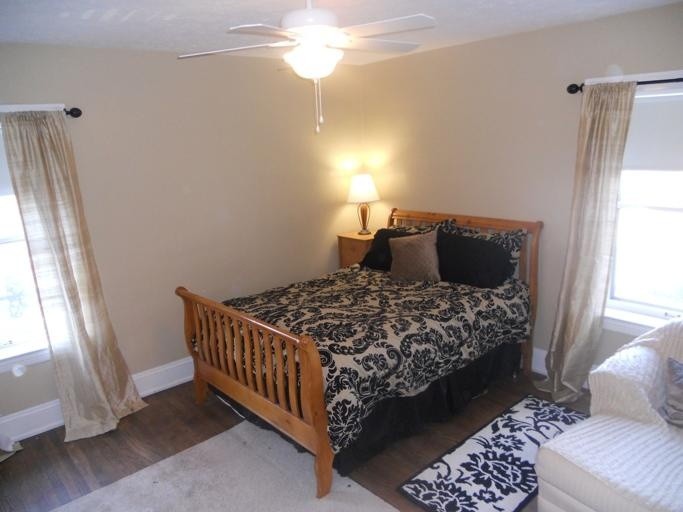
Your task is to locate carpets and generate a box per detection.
[50,418,399,512]
[396,393,590,512]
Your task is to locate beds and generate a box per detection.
[176,206,544,499]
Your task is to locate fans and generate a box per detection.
[176,0,438,60]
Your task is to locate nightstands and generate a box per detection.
[337,230,375,268]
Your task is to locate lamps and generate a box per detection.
[347,174,380,235]
[284,45,345,134]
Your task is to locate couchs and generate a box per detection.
[534,319,683,512]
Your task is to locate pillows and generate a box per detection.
[387,218,454,234]
[657,355,683,429]
[360,227,508,289]
[386,229,442,283]
[437,219,528,281]
